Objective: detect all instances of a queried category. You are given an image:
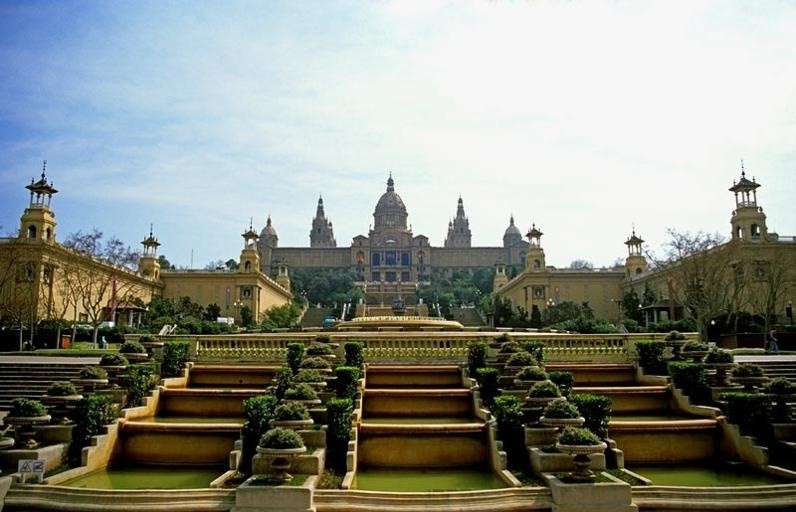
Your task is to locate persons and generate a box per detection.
[768,328,778,353]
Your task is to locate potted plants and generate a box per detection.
[487,332,609,458]
[3,333,164,424]
[256,334,340,459]
[663,326,796,423]
